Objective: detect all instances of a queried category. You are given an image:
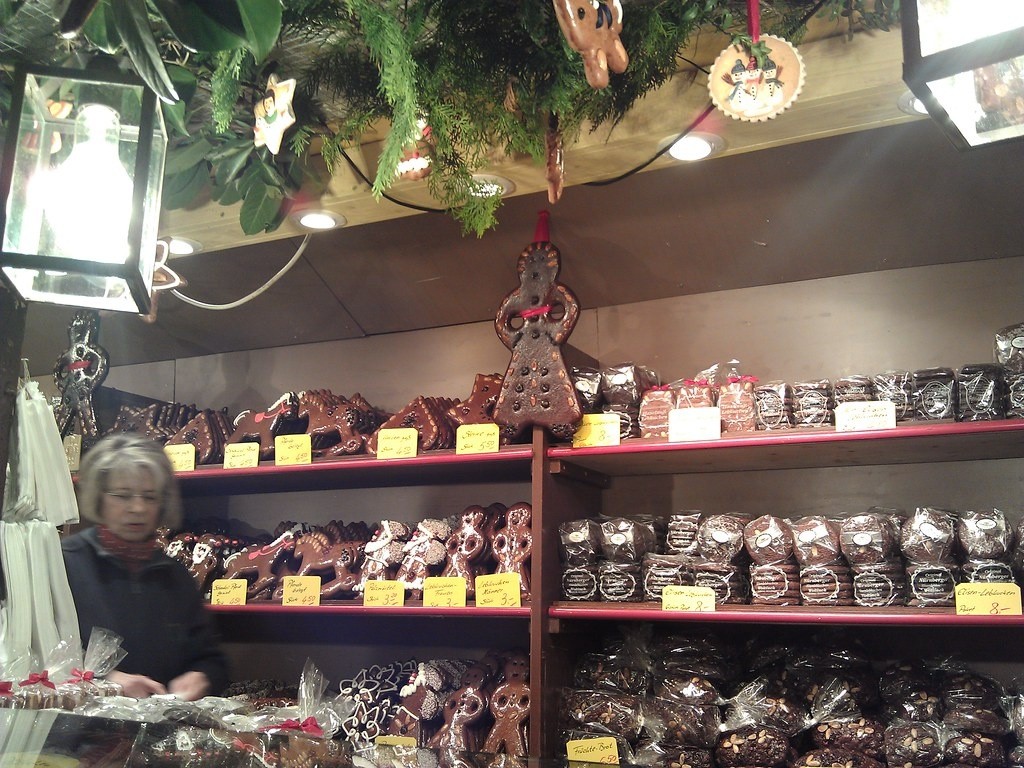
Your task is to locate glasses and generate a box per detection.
[103,489,164,508]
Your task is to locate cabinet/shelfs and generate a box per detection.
[71,338,1024,768]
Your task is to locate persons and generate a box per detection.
[60,434,226,700]
